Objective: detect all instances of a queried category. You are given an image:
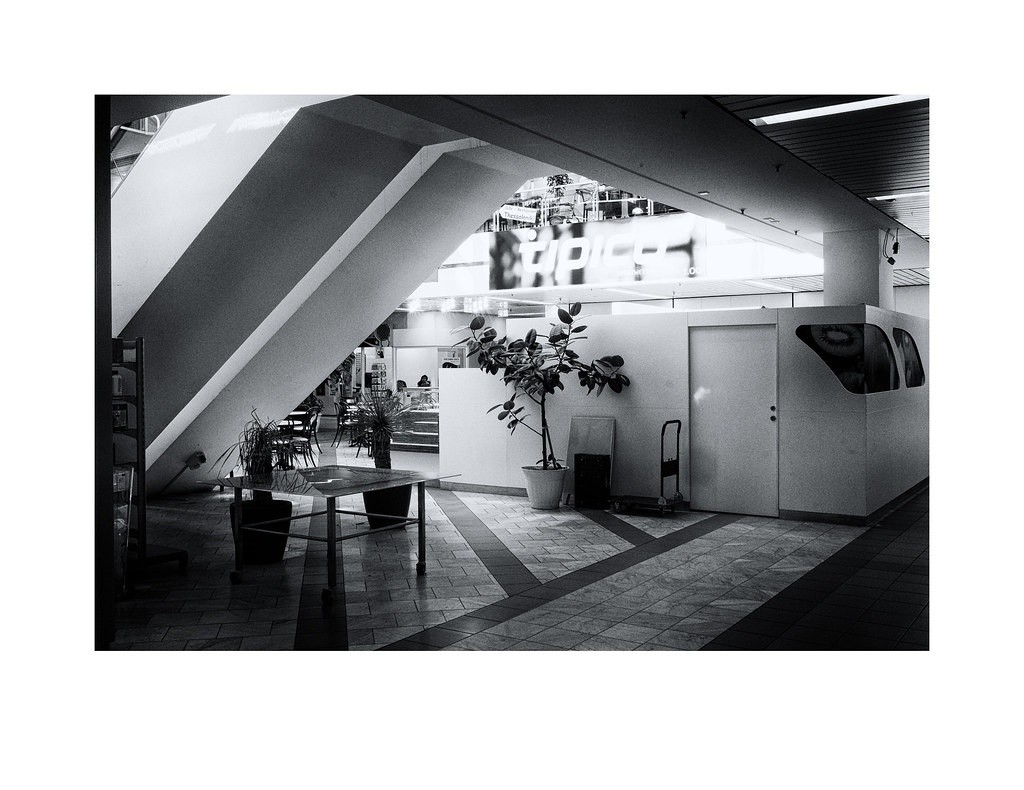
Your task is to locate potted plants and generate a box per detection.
[347,385,413,532]
[206,405,317,566]
[450,301,630,511]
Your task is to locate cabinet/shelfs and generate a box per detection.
[111,337,149,585]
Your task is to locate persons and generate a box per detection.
[418,375,431,395]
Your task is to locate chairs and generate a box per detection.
[575,188,606,221]
[520,196,543,228]
[276,401,373,468]
[499,202,523,231]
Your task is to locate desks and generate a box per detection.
[284,413,316,427]
[197,465,463,607]
